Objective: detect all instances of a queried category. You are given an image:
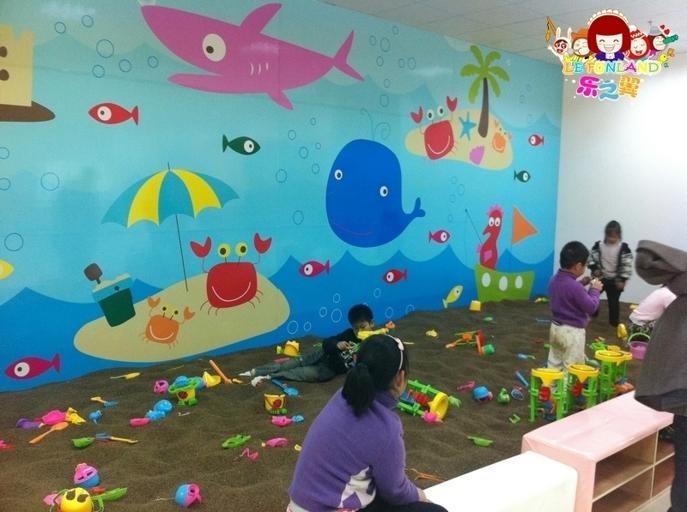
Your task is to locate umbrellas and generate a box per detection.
[98,158,241,295]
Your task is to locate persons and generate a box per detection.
[240,304,375,387]
[585,218,634,329]
[627,279,679,340]
[629,239,687,512]
[541,240,606,423]
[284,333,450,512]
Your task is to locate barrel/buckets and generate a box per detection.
[628,333,650,360]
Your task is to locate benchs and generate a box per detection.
[423,451,577,512]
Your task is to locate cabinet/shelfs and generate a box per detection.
[521,390,675,512]
